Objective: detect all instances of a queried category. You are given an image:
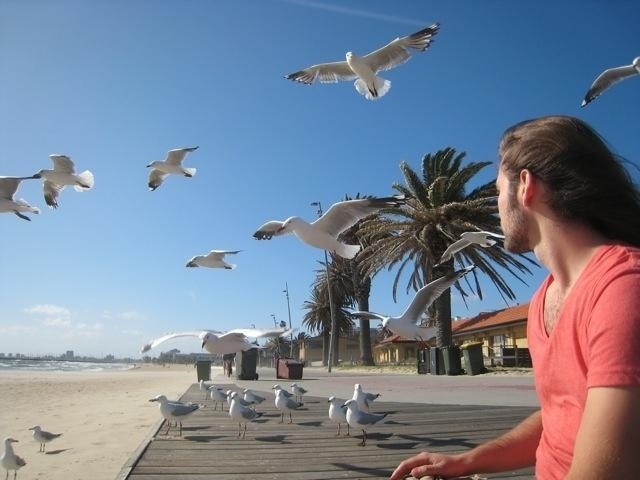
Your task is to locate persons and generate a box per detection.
[391,115,640,480]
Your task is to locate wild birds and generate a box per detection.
[580,56,640,115]
[144,146,197,192]
[148,377,392,443]
[0,150,95,222]
[338,226,506,344]
[280,22,440,102]
[184,196,408,270]
[137,325,297,352]
[0,422,64,480]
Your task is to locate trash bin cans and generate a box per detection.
[276,357,303,379]
[416,340,488,376]
[235,347,259,380]
[196,360,214,382]
